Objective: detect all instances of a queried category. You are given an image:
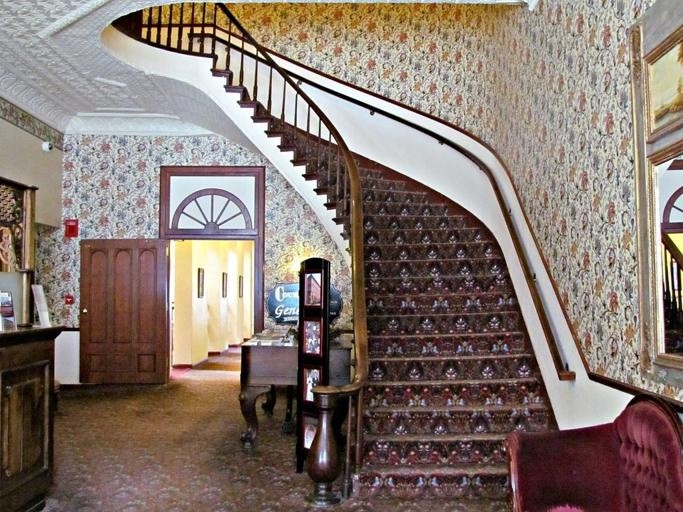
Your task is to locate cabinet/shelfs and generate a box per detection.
[1,325,68,511]
[237,323,353,450]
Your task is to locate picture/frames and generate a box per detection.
[238,275,243,297]
[222,272,227,298]
[198,267,204,298]
[622,16,681,373]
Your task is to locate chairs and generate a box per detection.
[502,391,681,512]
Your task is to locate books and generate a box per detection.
[247,336,284,342]
